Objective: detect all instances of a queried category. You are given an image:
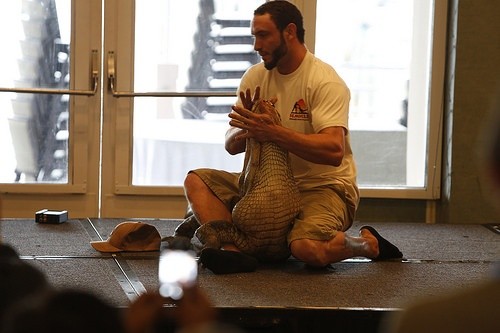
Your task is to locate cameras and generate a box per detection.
[35,208,68,224]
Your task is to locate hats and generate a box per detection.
[90,221,161,252]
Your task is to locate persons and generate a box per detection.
[0,97,500,333]
[184,0,403,275]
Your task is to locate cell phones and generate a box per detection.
[159,249,198,302]
